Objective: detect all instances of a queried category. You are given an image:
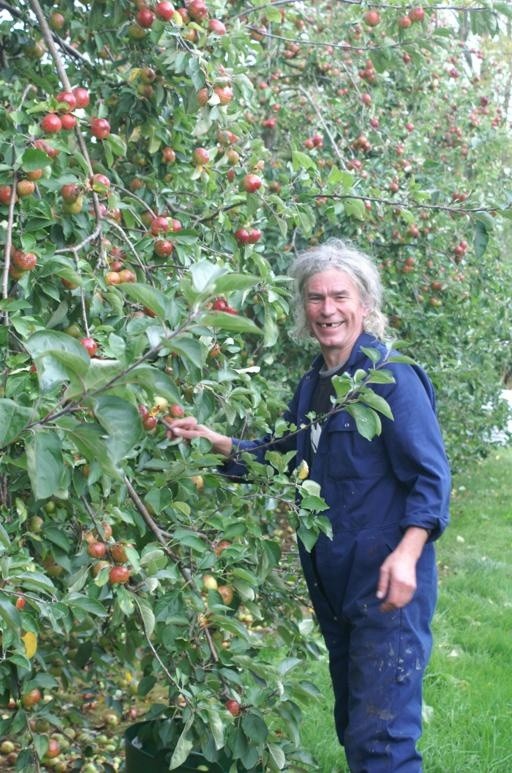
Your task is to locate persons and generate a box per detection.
[168,236,453,771]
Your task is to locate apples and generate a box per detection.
[2,0,504,773]
